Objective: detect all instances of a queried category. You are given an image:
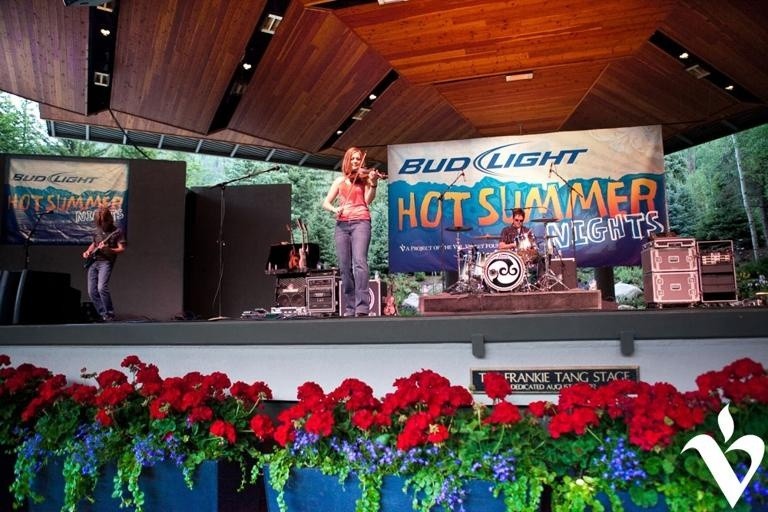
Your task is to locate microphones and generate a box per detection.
[547,163,553,177]
[461,166,467,182]
[267,165,280,172]
[34,209,55,216]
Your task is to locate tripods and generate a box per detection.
[449,252,568,293]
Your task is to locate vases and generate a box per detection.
[590,467,673,511]
[25,437,221,511]
[258,452,509,512]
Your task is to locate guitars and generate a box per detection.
[298,219,307,269]
[83,228,122,268]
[288,225,300,270]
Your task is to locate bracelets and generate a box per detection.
[370,185,377,188]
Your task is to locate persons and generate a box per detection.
[322,147,379,317]
[82,208,126,323]
[498,208,539,285]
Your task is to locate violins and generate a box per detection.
[349,166,390,183]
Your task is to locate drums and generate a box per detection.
[471,252,488,280]
[481,250,525,292]
[514,232,539,257]
[517,246,538,266]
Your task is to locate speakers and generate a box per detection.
[338,279,387,317]
[594,266,616,302]
[272,274,306,307]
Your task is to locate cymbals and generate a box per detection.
[530,219,558,222]
[473,235,502,239]
[445,226,472,231]
[535,235,557,238]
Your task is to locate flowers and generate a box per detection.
[548,358,766,511]
[263,363,547,512]
[0,354,272,511]
